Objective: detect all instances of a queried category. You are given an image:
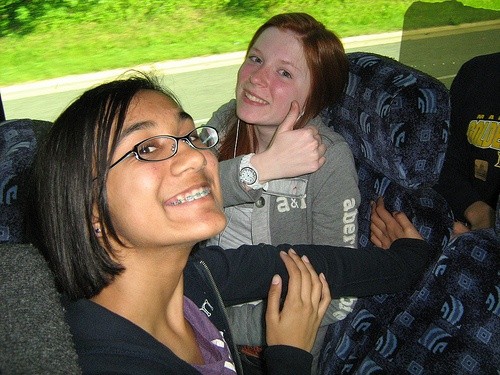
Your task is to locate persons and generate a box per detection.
[436,49,500,235]
[197,12,361,375]
[32,74,431,375]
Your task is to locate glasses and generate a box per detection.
[110,126,219,171]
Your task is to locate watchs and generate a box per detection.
[239,153,265,189]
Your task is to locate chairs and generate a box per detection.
[0,52,500,375]
